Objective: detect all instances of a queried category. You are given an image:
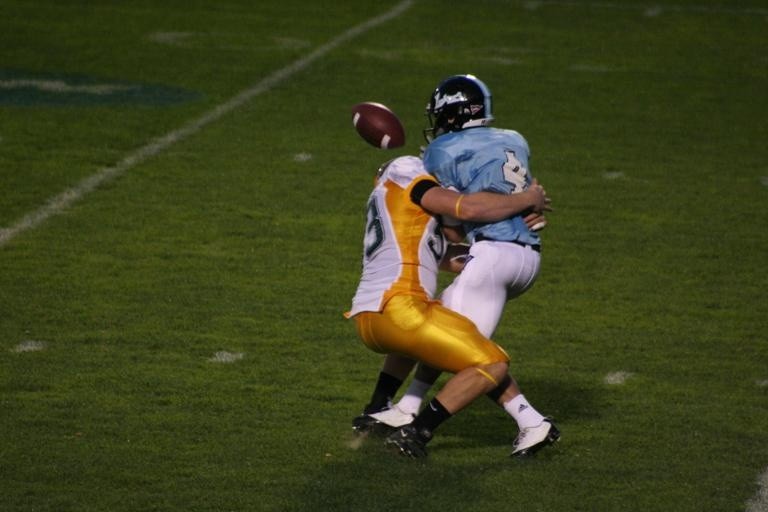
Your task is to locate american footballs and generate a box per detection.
[351,102,405,149]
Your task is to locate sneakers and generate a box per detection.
[352,402,394,431]
[363,407,415,432]
[509,418,560,460]
[386,426,428,461]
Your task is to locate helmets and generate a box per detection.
[423,75,494,143]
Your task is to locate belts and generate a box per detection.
[474,233,541,253]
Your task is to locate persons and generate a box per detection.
[339,155,553,460]
[351,72,560,460]
